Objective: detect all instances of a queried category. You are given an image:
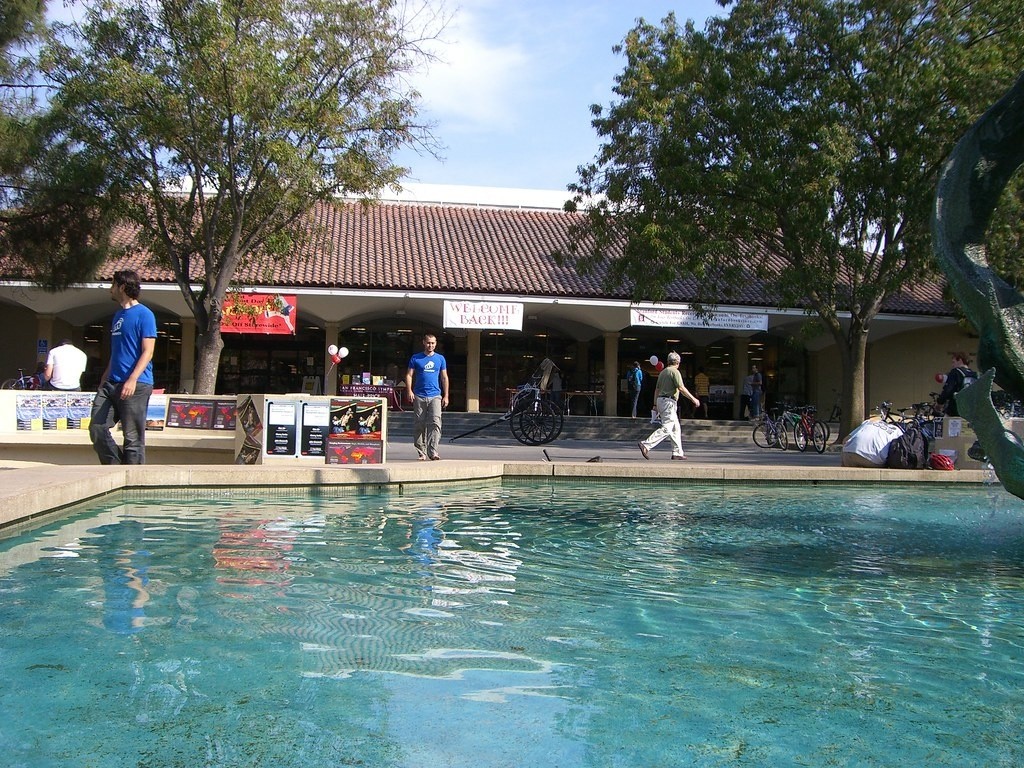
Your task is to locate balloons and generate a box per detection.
[656,361,664,372]
[650,355,658,366]
[328,345,338,356]
[338,347,349,358]
[332,354,341,364]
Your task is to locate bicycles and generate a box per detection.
[0,368,32,390]
[829,389,841,423]
[752,397,831,454]
[871,391,1022,439]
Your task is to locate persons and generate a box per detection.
[39,333,88,392]
[637,349,700,460]
[405,334,449,460]
[88,271,157,466]
[691,366,710,419]
[841,417,904,468]
[746,365,763,421]
[626,361,642,419]
[933,351,979,417]
[549,369,570,417]
[739,373,755,420]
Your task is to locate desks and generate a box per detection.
[507,388,550,415]
[341,384,406,413]
[563,392,603,416]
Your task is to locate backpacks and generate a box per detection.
[628,369,638,391]
[928,453,954,470]
[954,367,979,392]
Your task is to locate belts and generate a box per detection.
[658,395,676,402]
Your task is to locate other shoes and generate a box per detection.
[430,455,440,460]
[417,455,429,461]
[740,416,749,421]
[632,416,637,419]
[638,442,649,460]
[671,455,688,460]
[750,417,760,421]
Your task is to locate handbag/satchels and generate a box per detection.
[885,426,928,469]
[650,409,662,425]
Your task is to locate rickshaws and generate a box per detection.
[446,358,564,447]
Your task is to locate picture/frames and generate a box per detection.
[325,437,383,464]
[213,400,236,430]
[165,398,215,430]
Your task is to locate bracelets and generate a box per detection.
[654,404,656,406]
